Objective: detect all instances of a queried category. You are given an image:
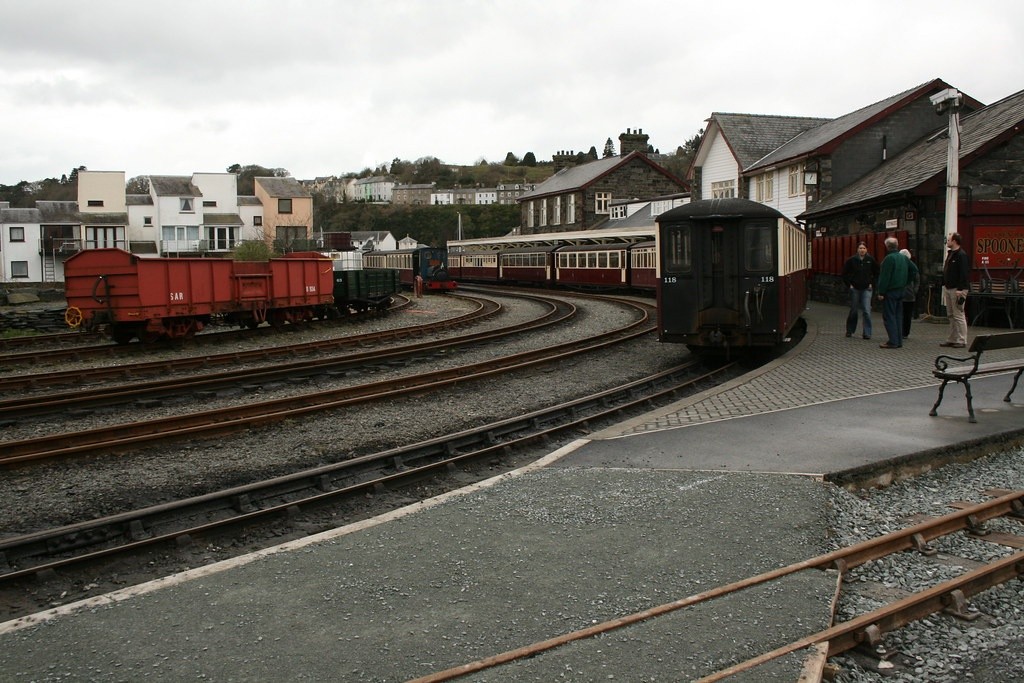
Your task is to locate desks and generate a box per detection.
[967,294,1024,330]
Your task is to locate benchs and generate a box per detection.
[970,259,1024,294]
[929,331,1024,424]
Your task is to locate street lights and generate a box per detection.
[457,211,463,241]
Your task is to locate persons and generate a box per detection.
[898,248,919,338]
[875,237,919,348]
[845,241,880,339]
[939,232,972,348]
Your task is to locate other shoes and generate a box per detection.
[940,342,954,347]
[879,343,898,348]
[953,344,966,347]
[863,336,871,339]
[846,331,851,337]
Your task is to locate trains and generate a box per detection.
[447,197,809,357]
[59,246,403,347]
[361,244,458,293]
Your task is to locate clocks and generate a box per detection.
[803,170,818,185]
